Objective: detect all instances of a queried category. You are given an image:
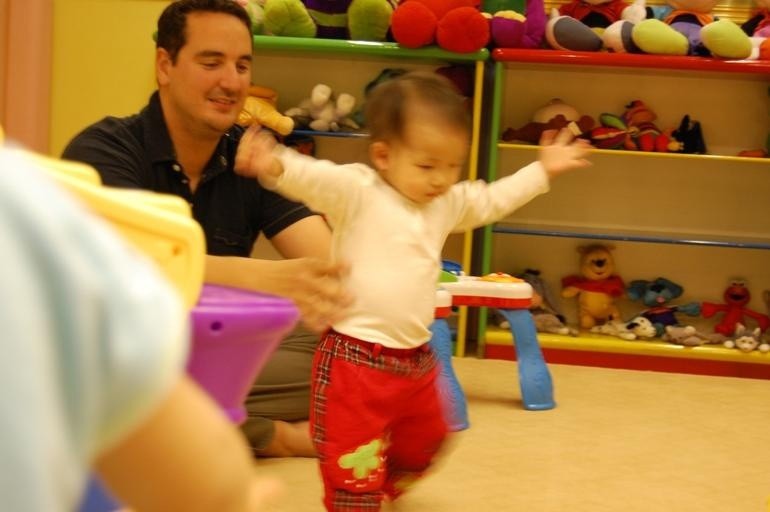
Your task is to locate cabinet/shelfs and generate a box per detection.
[152,31,492,357]
[475,48,770,381]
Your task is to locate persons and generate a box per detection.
[0,144,255,510]
[235,71,592,512]
[60,0,354,460]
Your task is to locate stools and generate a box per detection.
[428,289,470,430]
[441,274,554,411]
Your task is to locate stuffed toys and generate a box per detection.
[663,323,725,348]
[392,0,770,60]
[559,242,623,328]
[501,268,573,335]
[244,0,398,41]
[722,325,768,353]
[701,277,768,335]
[592,314,656,341]
[279,84,358,133]
[502,96,592,149]
[592,100,708,153]
[628,278,682,336]
[231,86,294,137]
